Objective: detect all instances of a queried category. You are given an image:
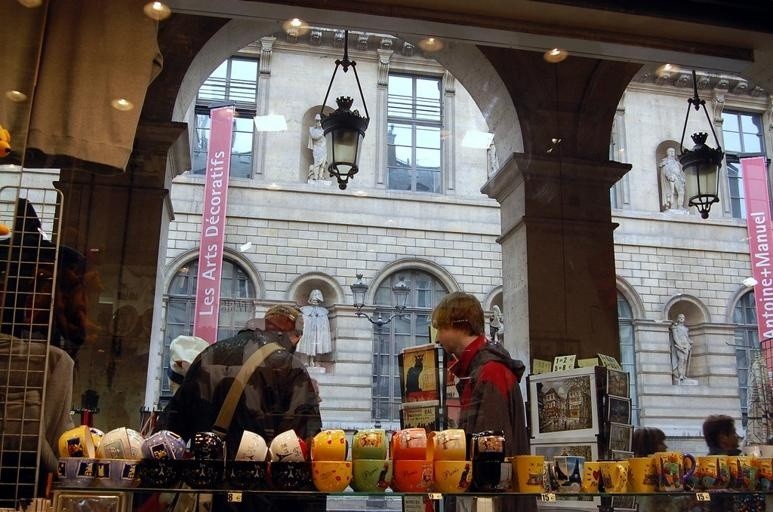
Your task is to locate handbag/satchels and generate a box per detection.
[168,478,216,512]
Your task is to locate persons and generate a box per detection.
[671,312,695,380]
[700,415,744,512]
[292,288,332,357]
[658,147,690,211]
[152,301,331,510]
[632,427,697,512]
[308,111,330,181]
[430,293,537,510]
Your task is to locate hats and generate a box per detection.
[263,302,305,332]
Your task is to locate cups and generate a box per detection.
[514,453,773,494]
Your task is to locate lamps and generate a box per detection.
[675,69,724,221]
[110,96,134,113]
[349,272,409,326]
[318,28,370,192]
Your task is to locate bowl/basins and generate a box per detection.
[54,427,512,493]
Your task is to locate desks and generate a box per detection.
[49,481,772,512]
